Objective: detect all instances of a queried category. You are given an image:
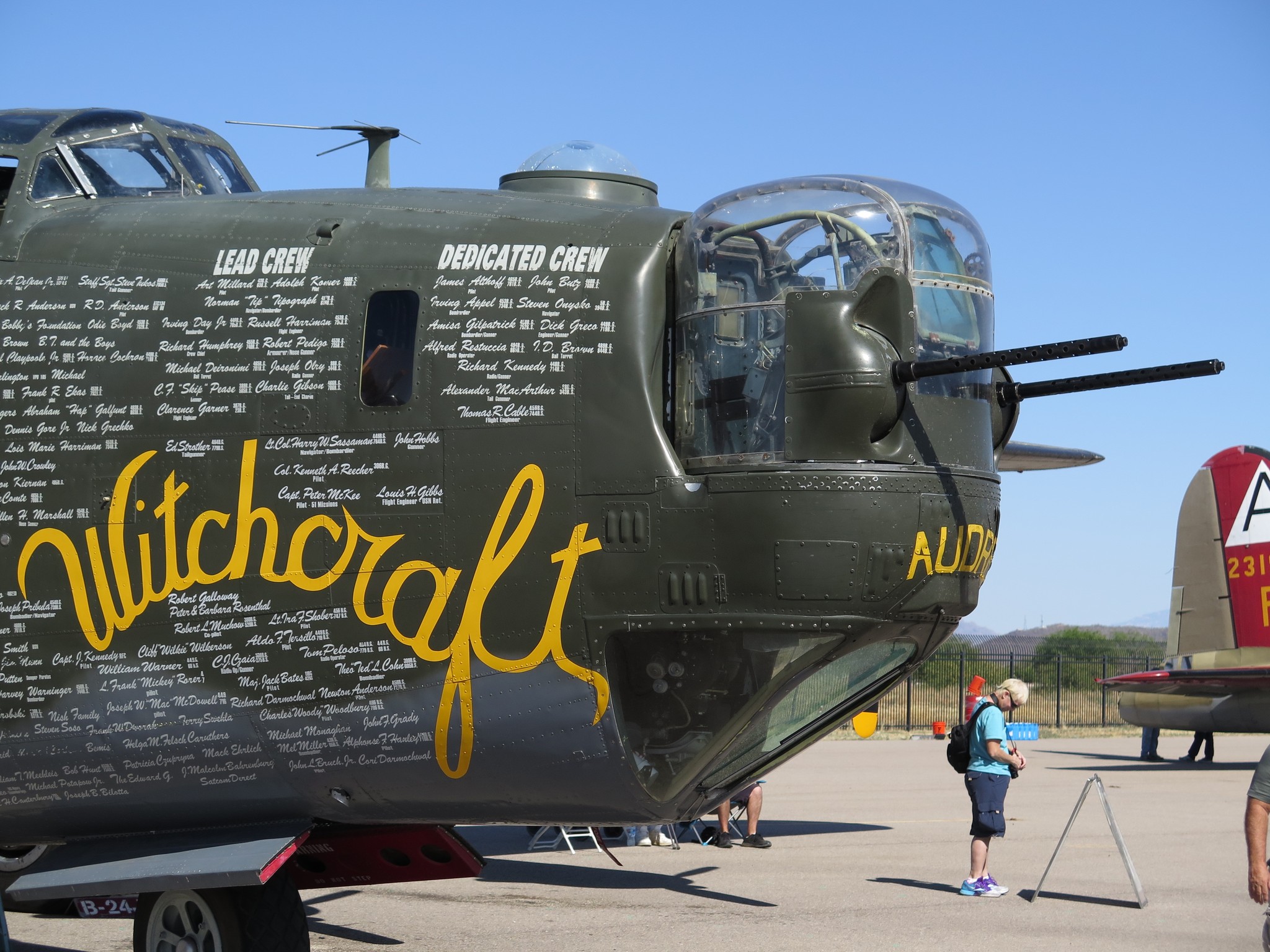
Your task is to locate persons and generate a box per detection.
[1140,667,1163,760]
[715,784,772,848]
[635,824,675,845]
[1244,744,1270,952]
[960,678,1030,897]
[1178,731,1214,762]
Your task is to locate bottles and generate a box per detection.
[1005,722,1039,740]
[627,826,635,846]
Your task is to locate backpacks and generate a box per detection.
[946,702,1000,774]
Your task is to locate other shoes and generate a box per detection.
[1198,758,1212,763]
[1149,753,1164,760]
[1179,755,1194,761]
[1139,755,1156,761]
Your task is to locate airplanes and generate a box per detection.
[1098,444,1270,735]
[0,104,1227,952]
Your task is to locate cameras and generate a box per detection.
[1008,751,1019,779]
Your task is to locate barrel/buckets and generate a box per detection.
[969,675,986,696]
[932,721,946,740]
[965,694,986,723]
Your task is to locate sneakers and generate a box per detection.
[648,824,674,846]
[635,825,652,846]
[984,873,1009,894]
[716,832,733,848]
[742,834,772,847]
[959,876,1001,897]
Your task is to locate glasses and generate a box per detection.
[1006,688,1018,709]
